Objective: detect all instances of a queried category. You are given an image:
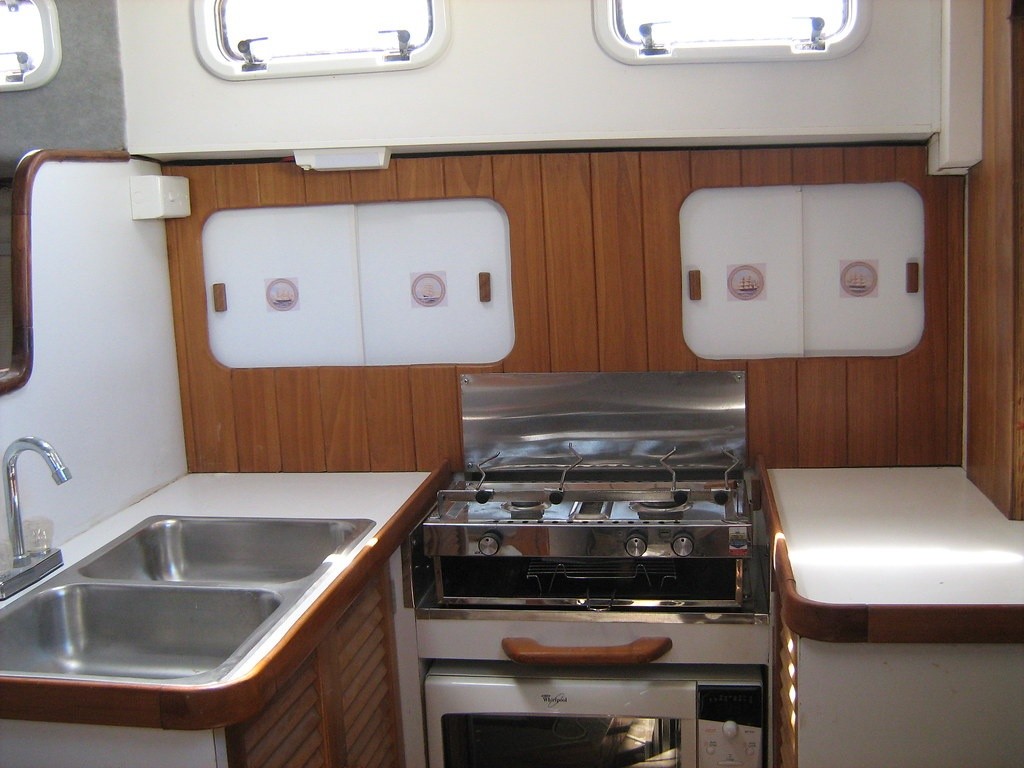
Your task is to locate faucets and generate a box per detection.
[2,436,72,567]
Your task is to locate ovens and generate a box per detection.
[423,658,764,768]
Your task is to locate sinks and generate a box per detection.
[77,516,379,584]
[0,584,281,679]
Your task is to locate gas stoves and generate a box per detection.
[421,369,754,609]
